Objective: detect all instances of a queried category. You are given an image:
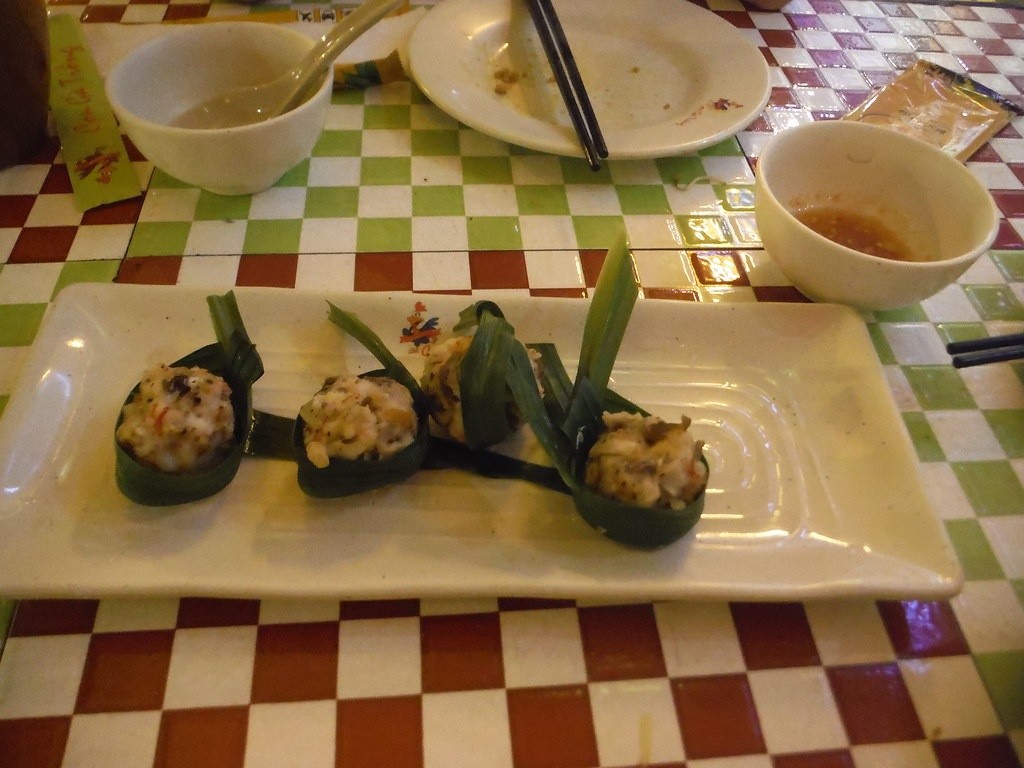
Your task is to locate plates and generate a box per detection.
[408,0,770,161]
[0,280,968,601]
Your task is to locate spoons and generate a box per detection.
[166,0,411,129]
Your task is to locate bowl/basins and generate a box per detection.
[753,119,999,313]
[104,20,335,197]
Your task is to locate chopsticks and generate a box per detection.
[521,0,609,173]
[944,332,1024,377]
